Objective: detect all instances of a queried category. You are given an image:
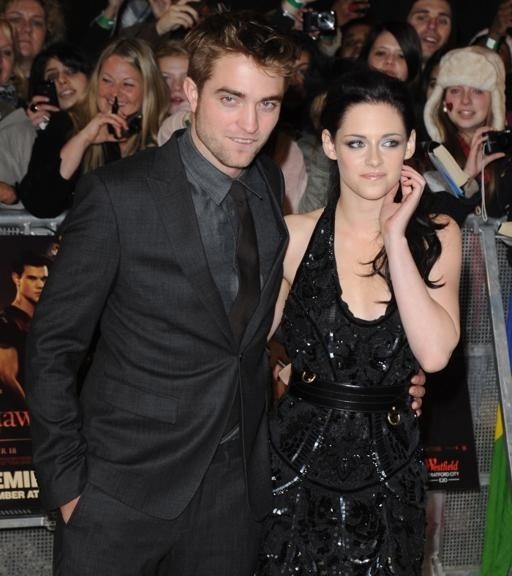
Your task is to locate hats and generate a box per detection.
[423,45,506,143]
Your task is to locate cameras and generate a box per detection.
[303,9,336,36]
[482,131,512,155]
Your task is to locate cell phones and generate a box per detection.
[121,110,142,138]
[36,80,58,106]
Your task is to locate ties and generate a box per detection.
[229,182,261,340]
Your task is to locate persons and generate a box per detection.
[23,15,429,575]
[269,70,464,576]
[0,255,51,398]
